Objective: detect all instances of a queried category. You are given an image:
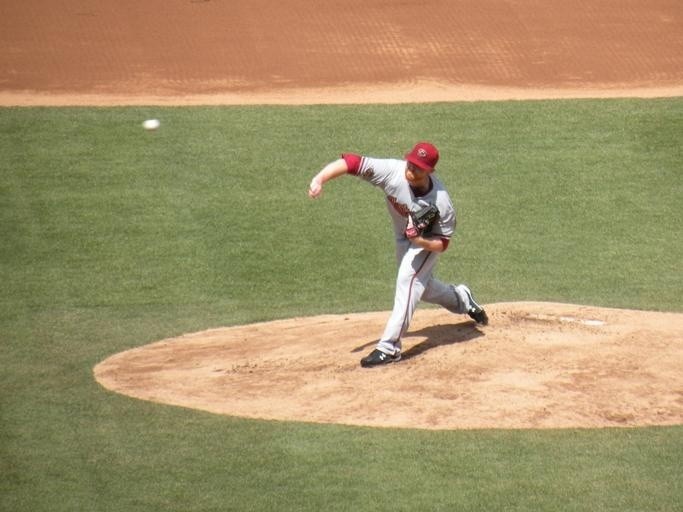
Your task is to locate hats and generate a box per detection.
[403,142,440,173]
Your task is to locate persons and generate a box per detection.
[308,142,488,368]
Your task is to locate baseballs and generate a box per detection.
[143,120,160,130]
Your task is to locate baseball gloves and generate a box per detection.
[411,201,440,232]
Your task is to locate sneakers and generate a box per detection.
[358,349,403,367]
[457,282,489,328]
